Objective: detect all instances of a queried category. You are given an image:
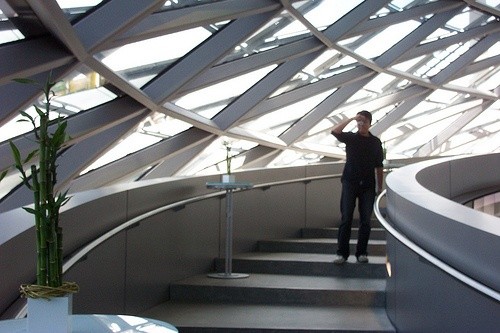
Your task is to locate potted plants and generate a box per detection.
[9,68,79,319]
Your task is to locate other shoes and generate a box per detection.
[334,256,345,264]
[357,255,368,262]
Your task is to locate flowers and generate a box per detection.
[223,138,242,174]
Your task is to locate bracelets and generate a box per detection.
[377,192,382,195]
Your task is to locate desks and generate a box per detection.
[2,314,177,333]
[206,180,254,280]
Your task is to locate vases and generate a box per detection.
[221,175,235,184]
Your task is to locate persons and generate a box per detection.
[330,110,384,264]
